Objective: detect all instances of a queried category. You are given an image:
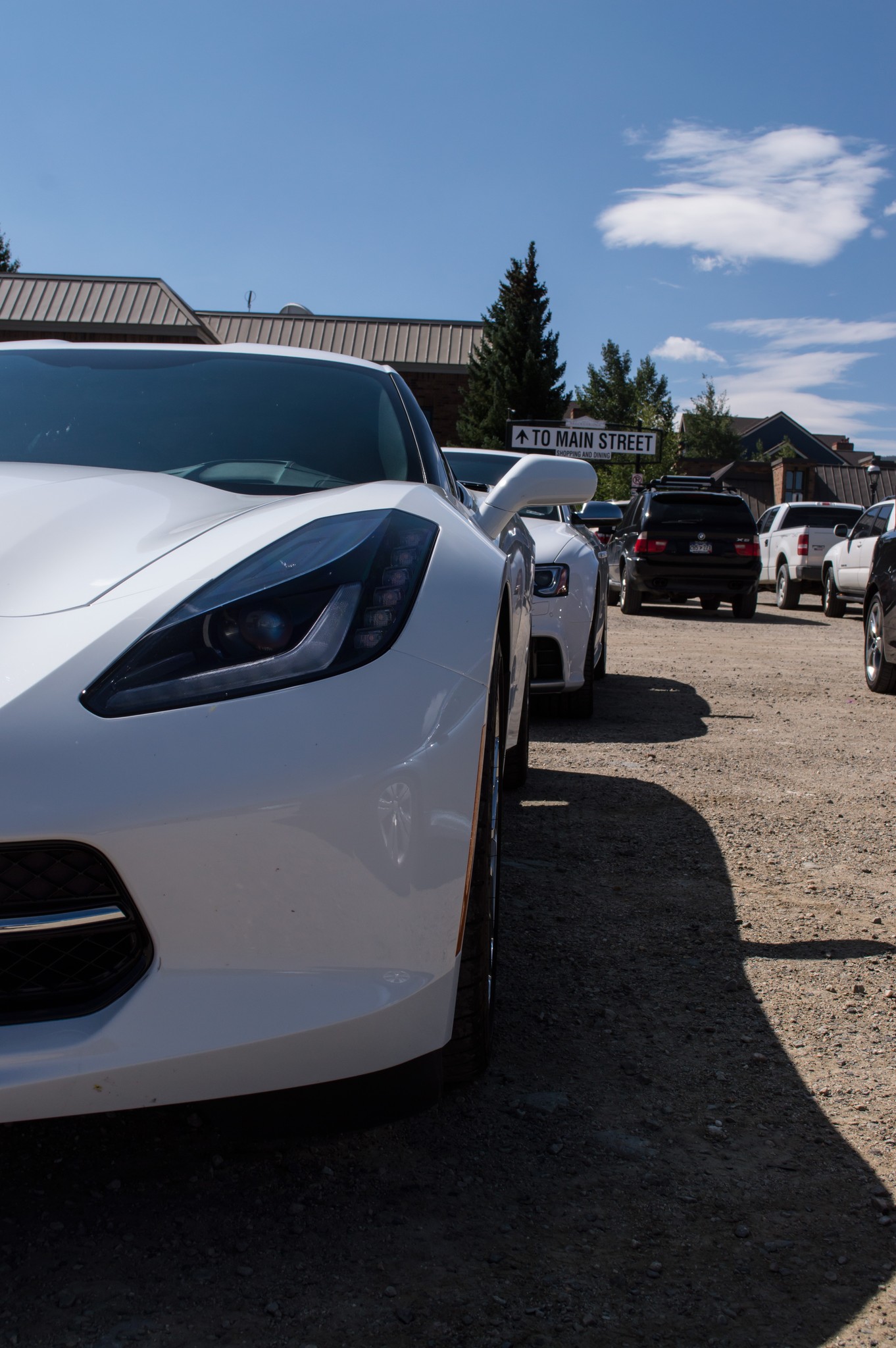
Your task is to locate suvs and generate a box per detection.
[600,474,762,620]
[819,500,896,619]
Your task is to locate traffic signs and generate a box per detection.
[509,424,656,459]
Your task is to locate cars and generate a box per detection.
[435,443,632,714]
[0,340,600,1130]
[586,502,634,544]
[864,521,896,691]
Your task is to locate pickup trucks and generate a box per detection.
[755,502,867,611]
